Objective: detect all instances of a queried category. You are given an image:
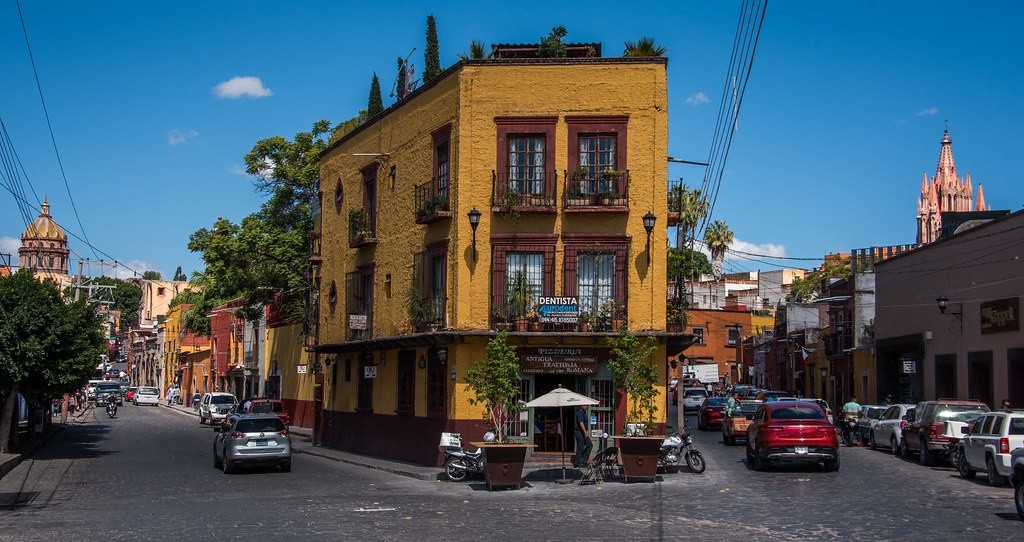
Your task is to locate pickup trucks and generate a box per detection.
[248,399,291,436]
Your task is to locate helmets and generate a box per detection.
[483,432,495,442]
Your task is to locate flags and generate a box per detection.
[801,346,810,360]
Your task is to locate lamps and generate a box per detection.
[679,350,686,363]
[417,353,426,369]
[467,207,482,262]
[641,211,657,266]
[667,360,678,369]
[324,356,334,366]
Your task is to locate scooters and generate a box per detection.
[103,400,120,419]
[837,414,864,448]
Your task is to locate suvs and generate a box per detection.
[900,397,992,467]
[745,401,841,472]
[213,411,292,474]
[957,408,1024,487]
[198,391,241,426]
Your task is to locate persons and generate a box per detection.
[573,405,593,468]
[797,388,803,398]
[166,381,180,406]
[52,391,81,416]
[1002,399,1010,409]
[105,392,118,414]
[725,391,739,417]
[689,380,695,385]
[119,370,126,381]
[839,394,870,447]
[755,391,778,402]
[244,400,251,411]
[193,389,201,411]
[707,381,733,397]
[735,379,742,383]
[881,394,893,406]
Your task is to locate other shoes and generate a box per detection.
[573,461,581,467]
[582,463,590,467]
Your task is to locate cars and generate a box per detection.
[868,403,918,455]
[132,386,160,406]
[125,387,138,402]
[853,405,888,446]
[1009,446,1024,520]
[81,352,131,408]
[669,373,836,447]
[226,404,240,423]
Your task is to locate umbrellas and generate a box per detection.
[525,383,601,479]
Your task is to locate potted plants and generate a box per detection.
[597,191,627,205]
[491,304,515,331]
[567,166,591,205]
[506,270,535,332]
[463,333,539,491]
[578,308,600,332]
[493,187,522,228]
[603,303,623,331]
[425,195,449,211]
[529,193,555,206]
[607,319,666,483]
[404,287,442,333]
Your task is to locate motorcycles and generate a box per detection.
[439,428,500,482]
[657,417,707,474]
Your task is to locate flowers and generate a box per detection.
[666,305,693,329]
[528,307,540,322]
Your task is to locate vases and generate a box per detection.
[666,321,680,332]
[530,321,544,332]
